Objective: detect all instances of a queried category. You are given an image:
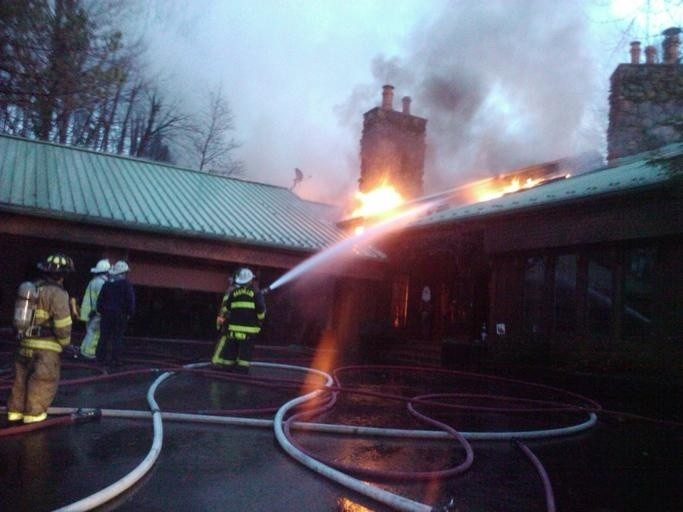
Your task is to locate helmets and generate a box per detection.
[89,259,110,274]
[36,251,76,274]
[109,260,130,276]
[235,268,254,284]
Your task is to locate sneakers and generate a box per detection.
[7,410,48,424]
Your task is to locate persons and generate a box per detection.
[215,266,268,376]
[72,258,113,363]
[93,258,137,368]
[4,251,78,426]
